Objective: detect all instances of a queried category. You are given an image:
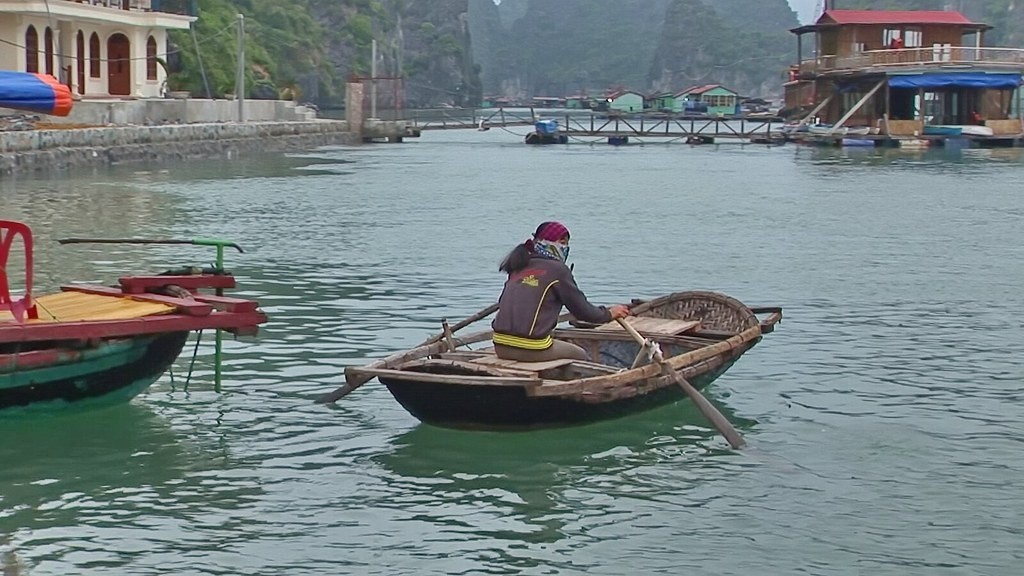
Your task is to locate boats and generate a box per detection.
[924,125,963,137]
[341,285,785,431]
[745,116,784,123]
[0,219,268,407]
[939,123,995,137]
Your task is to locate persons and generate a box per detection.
[491,221,629,363]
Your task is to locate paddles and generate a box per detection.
[614,315,747,450]
[312,302,500,404]
